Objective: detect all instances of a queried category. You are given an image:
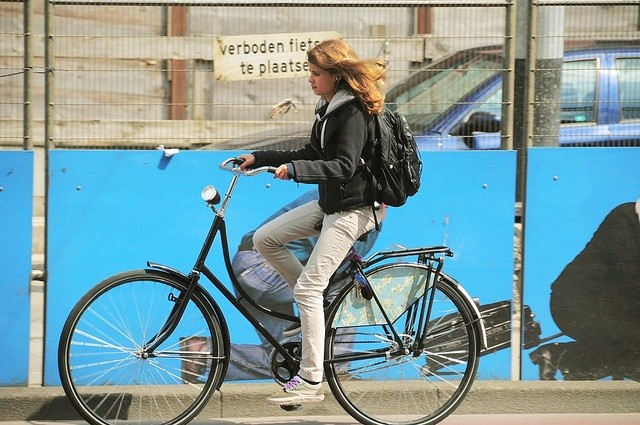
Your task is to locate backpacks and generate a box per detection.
[359,99,423,207]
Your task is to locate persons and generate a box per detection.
[531,200,638,383]
[181,190,382,385]
[235,37,390,405]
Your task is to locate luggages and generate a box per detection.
[421,299,565,375]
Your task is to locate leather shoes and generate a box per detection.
[325,372,374,382]
[180,338,208,385]
[530,343,558,382]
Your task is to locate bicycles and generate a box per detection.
[57,157,487,425]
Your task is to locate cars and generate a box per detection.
[196,38,636,148]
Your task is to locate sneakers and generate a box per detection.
[266,376,324,406]
[283,318,301,337]
